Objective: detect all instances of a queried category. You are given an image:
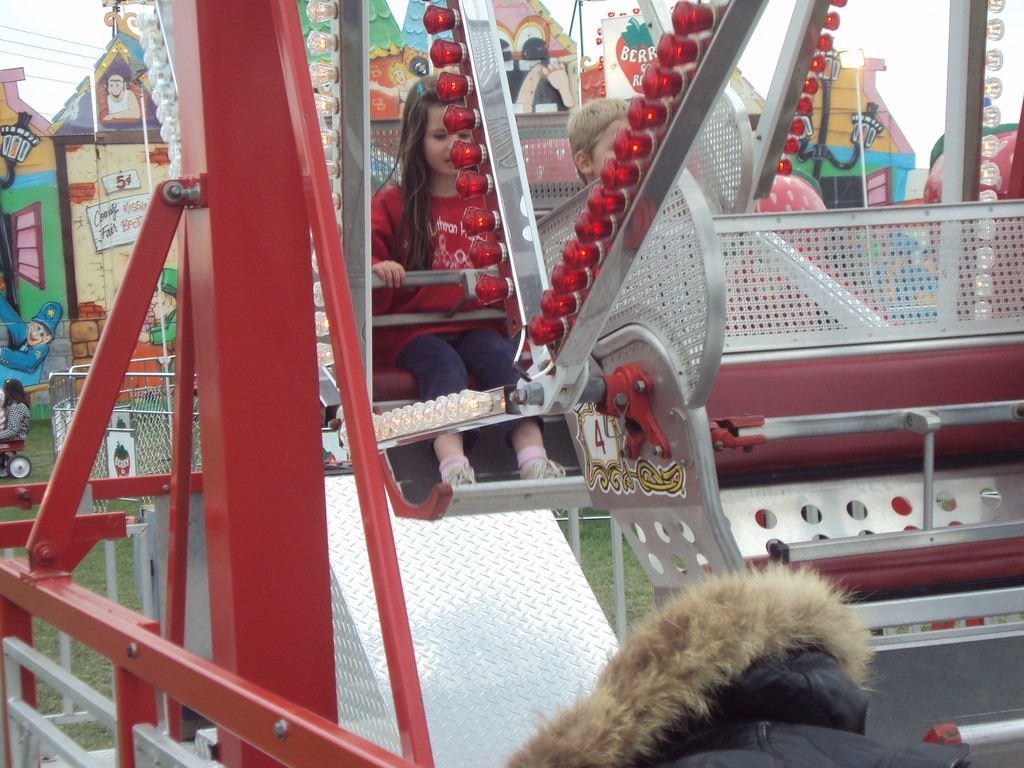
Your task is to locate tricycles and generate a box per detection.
[0,439,32,479]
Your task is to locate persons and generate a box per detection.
[0,379,30,479]
[569,98,630,186]
[372,77,568,487]
[509,564,977,768]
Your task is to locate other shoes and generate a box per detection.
[518,458,567,479]
[443,462,479,487]
[0,468,9,478]
